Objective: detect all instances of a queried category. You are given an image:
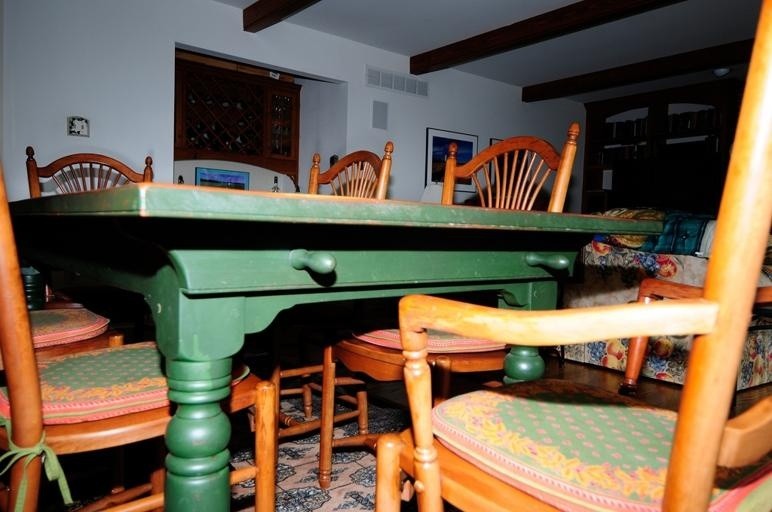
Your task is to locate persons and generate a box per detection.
[454,149,550,212]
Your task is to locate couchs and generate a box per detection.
[550,209,772,392]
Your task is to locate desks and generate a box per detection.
[8,181,664,510]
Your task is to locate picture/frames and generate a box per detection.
[67,116,89,137]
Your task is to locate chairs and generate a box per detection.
[373,2,772,511]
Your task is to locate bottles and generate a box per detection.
[270,175,280,193]
